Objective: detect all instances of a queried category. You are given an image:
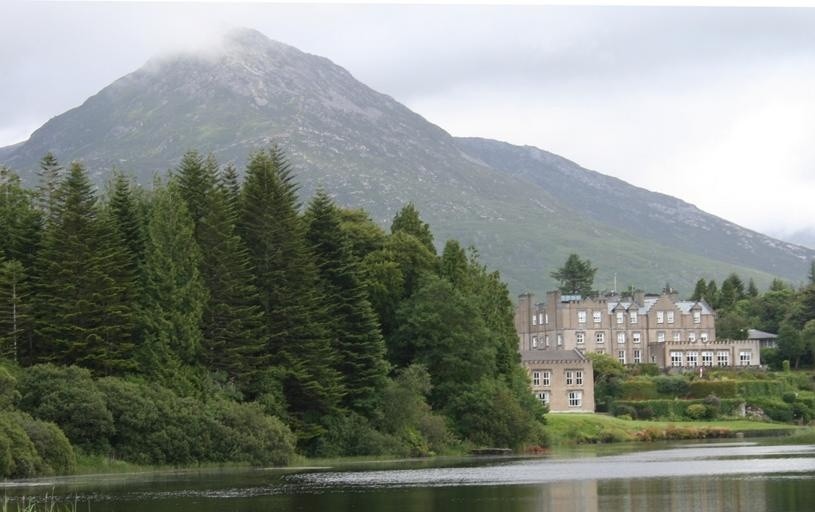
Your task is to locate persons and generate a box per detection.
[698,365,703,380]
